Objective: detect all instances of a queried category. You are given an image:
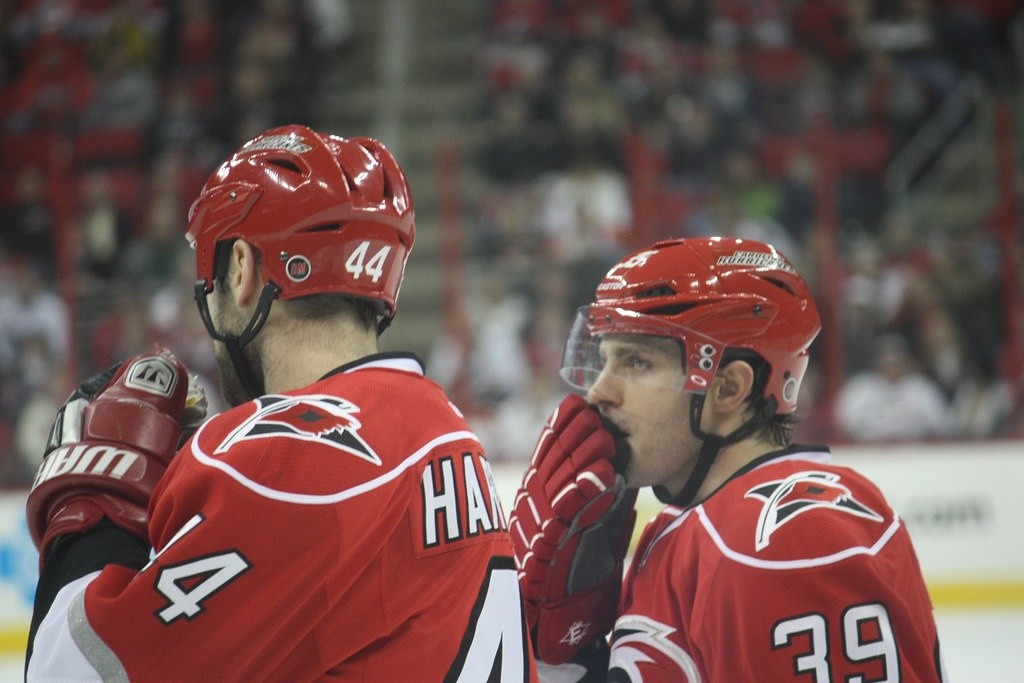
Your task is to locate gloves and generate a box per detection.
[26,346,206,564]
[509,392,638,666]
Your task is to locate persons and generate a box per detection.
[510,238,946,683]
[1,0,1023,473]
[26,125,539,683]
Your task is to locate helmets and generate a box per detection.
[587,238,821,417]
[184,126,415,319]
